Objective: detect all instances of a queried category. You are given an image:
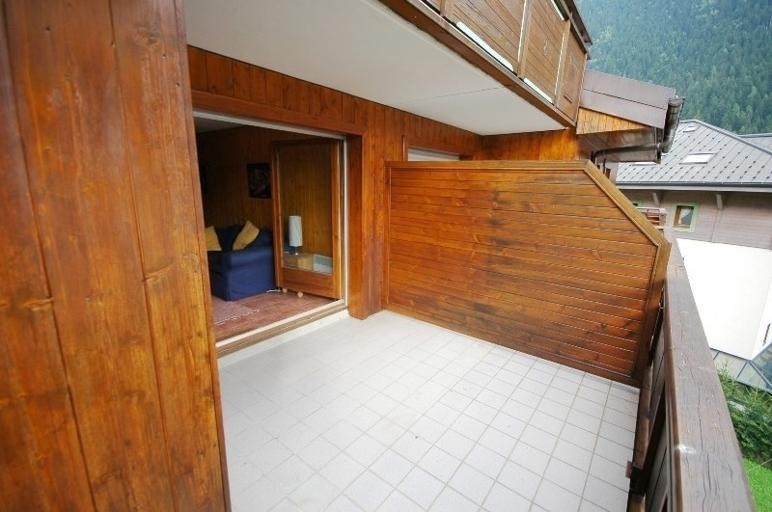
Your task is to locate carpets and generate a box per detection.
[210,289,340,345]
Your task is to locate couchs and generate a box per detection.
[207,227,298,301]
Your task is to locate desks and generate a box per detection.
[281,252,314,270]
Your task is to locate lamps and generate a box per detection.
[288,215,303,255]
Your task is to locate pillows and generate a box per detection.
[205,219,261,253]
